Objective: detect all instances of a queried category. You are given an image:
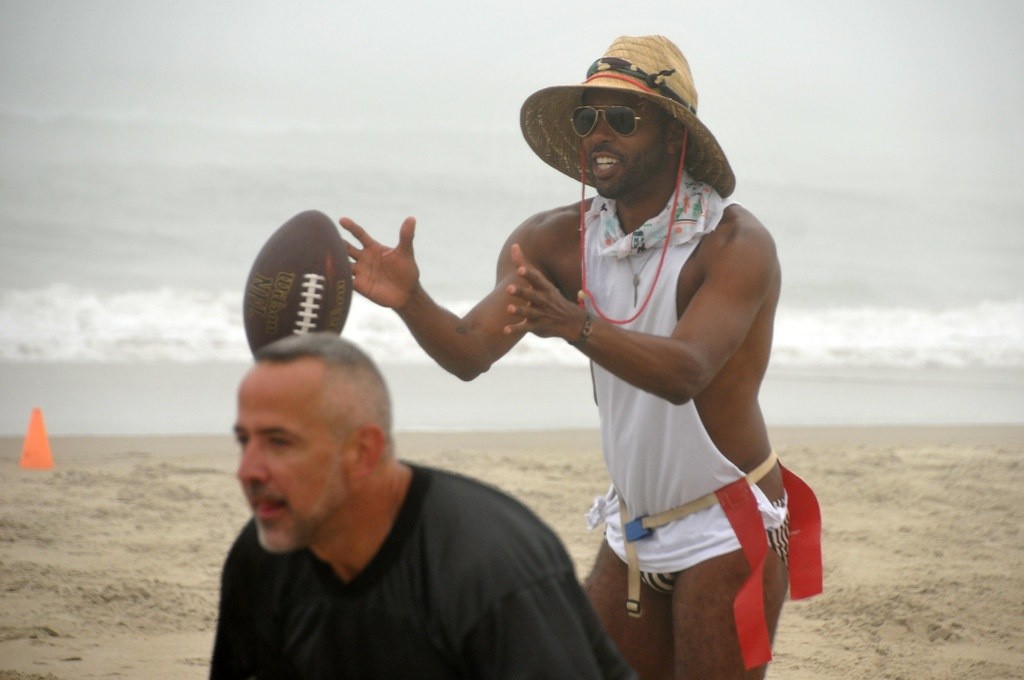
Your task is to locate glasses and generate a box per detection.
[570,105,668,138]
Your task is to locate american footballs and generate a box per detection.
[241,208,353,357]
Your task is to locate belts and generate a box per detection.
[616,452,778,619]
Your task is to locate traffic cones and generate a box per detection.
[17,407,53,468]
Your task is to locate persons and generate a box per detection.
[342,34,791,680]
[208,331,641,680]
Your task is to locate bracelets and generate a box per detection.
[567,311,593,346]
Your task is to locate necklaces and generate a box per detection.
[621,247,656,307]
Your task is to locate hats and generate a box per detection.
[519,35,737,199]
